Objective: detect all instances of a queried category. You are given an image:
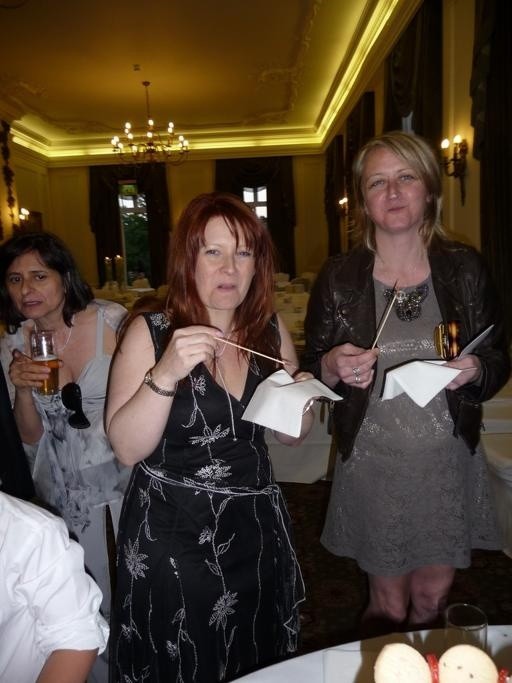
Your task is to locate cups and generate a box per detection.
[445,602,487,650]
[27,329,61,399]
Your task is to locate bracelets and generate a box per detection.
[302,398,314,418]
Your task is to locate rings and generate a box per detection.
[352,367,360,374]
[355,376,360,383]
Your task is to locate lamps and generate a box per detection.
[338,197,347,211]
[110,118,189,172]
[439,136,470,204]
[18,208,33,227]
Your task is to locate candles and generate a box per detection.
[116,255,126,283]
[105,256,112,279]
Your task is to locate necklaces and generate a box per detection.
[376,246,423,287]
[202,322,236,358]
[33,315,75,354]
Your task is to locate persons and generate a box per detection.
[1,489,110,683]
[104,191,316,682]
[0,230,132,624]
[301,135,512,635]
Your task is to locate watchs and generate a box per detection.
[144,368,179,397]
